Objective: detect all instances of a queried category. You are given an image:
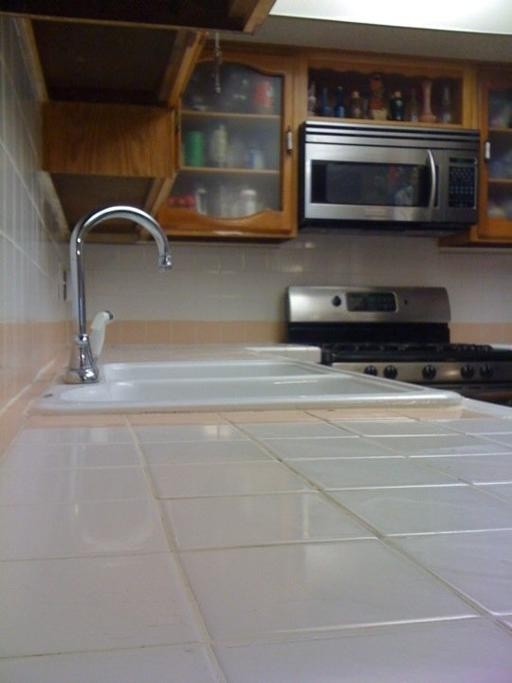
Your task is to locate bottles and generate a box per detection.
[185,130,208,167]
[307,80,456,124]
[207,121,229,168]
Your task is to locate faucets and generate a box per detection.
[63,202,175,382]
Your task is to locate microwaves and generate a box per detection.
[297,120,481,240]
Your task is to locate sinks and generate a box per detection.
[99,356,348,378]
[47,372,461,410]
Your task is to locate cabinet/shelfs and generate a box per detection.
[295,49,481,130]
[152,41,300,244]
[438,70,512,250]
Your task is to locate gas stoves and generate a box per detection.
[305,339,512,363]
